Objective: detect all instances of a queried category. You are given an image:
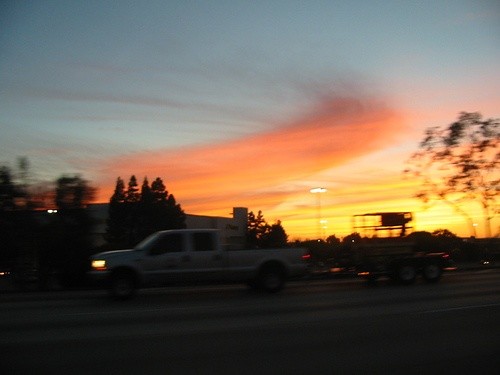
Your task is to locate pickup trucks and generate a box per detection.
[87,228,308,302]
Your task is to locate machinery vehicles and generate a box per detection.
[348,213,450,286]
